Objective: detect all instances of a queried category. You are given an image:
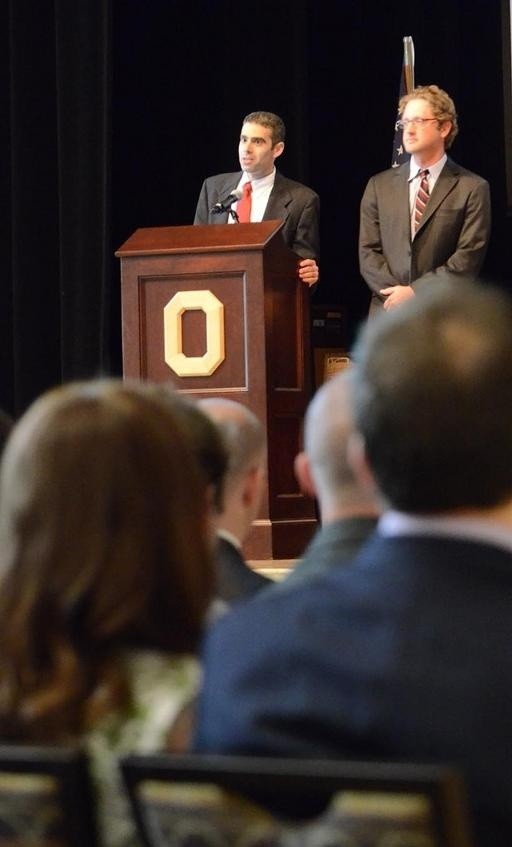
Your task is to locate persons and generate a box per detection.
[196,276,512,847]
[194,111,321,297]
[0,377,228,847]
[358,85,491,326]
[274,371,386,592]
[192,398,276,612]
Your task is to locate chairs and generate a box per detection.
[118,750,470,847]
[0,743,99,845]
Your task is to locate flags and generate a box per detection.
[390,37,416,168]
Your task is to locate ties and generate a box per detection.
[235,182,252,223]
[415,171,430,231]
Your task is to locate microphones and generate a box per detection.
[212,189,242,215]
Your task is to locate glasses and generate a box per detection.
[396,117,436,130]
